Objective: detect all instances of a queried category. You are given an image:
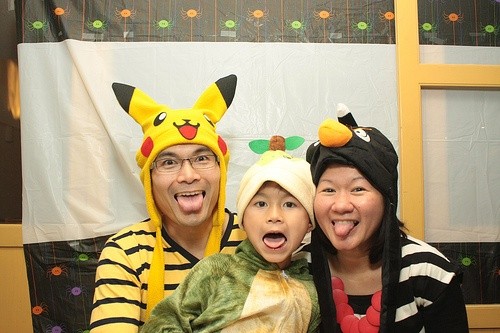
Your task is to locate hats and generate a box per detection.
[236,136,315,232]
[111,74,237,321]
[306,104,402,333]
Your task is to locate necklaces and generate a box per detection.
[330,275,382,333]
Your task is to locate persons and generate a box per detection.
[89,74,246,333]
[291,103,469,333]
[141,135,321,333]
